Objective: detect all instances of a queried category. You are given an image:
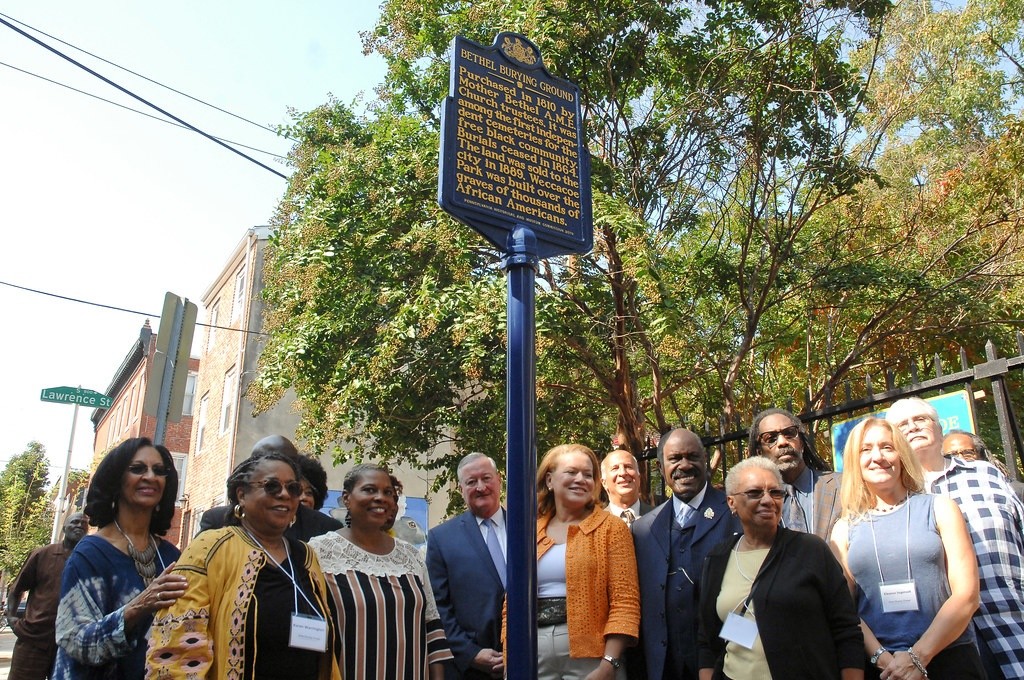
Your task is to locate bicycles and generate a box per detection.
[0,610,7,634]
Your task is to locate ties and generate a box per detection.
[623,510,635,528]
[483,519,507,591]
[678,506,694,526]
[785,485,807,532]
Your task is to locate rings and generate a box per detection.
[156,593,161,601]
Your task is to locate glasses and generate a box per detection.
[731,489,788,499]
[756,425,800,444]
[128,461,172,476]
[242,479,304,498]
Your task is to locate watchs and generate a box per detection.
[602,654,621,670]
[870,647,887,664]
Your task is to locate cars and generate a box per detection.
[16,600,28,618]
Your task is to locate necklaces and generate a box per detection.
[112,518,157,590]
[871,491,913,514]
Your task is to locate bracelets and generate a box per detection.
[906,647,929,675]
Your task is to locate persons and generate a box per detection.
[194,434,343,556]
[361,467,402,538]
[499,441,642,680]
[939,429,1024,501]
[627,426,743,680]
[693,451,867,680]
[144,453,344,680]
[744,407,846,554]
[305,462,455,680]
[596,451,657,544]
[295,452,329,511]
[426,451,508,680]
[7,511,90,680]
[825,418,990,679]
[882,396,1024,680]
[49,434,184,680]
